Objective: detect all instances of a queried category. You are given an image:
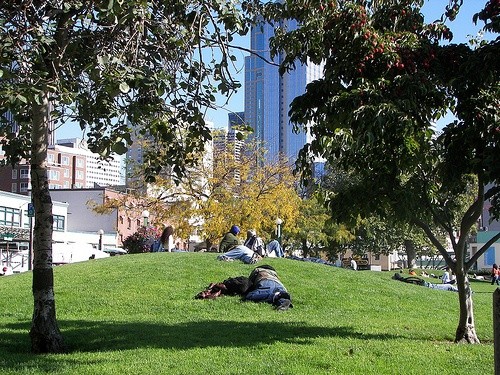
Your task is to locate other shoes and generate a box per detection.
[274,299,290,310]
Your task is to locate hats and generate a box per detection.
[230,225,242,233]
[408,268,415,274]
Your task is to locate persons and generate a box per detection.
[394,264,500,292]
[326,256,357,271]
[194,265,291,310]
[157,222,285,263]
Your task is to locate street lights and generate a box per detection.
[142,209,149,226]
[98,229,103,250]
[276,217,282,244]
